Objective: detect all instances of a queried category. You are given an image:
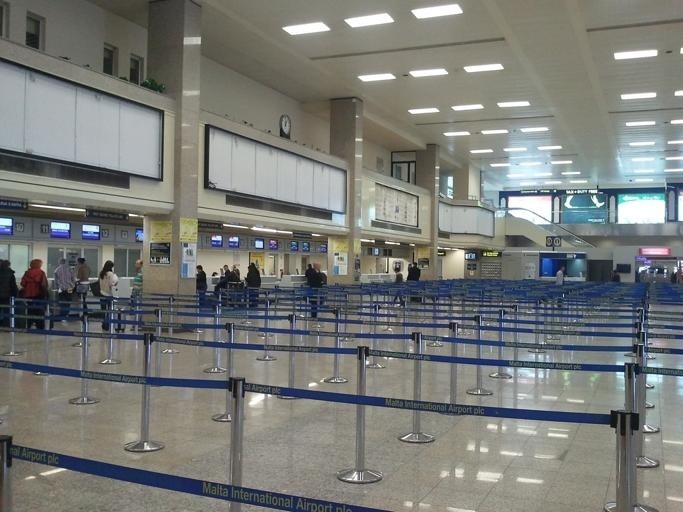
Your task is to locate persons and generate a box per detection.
[392,267,405,307]
[611,269,620,282]
[305,263,316,284]
[128,259,144,324]
[211,261,261,308]
[303,262,327,317]
[0,256,92,331]
[554,265,567,307]
[406,261,423,302]
[96,259,118,332]
[195,264,207,307]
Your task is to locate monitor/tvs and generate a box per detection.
[465,253,476,259]
[229,235,240,249]
[210,234,224,248]
[51,222,72,240]
[289,241,299,253]
[81,225,100,240]
[134,230,143,243]
[301,242,310,254]
[0,218,12,235]
[374,249,380,255]
[319,244,327,253]
[268,240,279,252]
[254,238,265,251]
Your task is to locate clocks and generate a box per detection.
[280,114,291,135]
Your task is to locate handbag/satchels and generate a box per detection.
[41,272,47,290]
[90,281,100,295]
